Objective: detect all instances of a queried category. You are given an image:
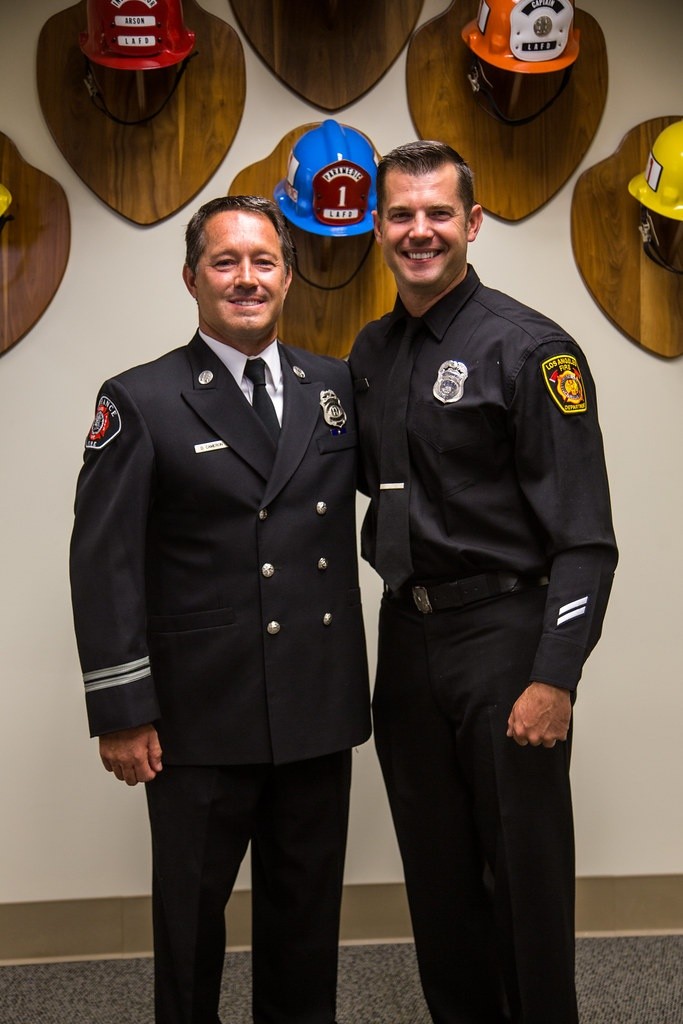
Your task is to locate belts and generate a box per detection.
[389,570,549,617]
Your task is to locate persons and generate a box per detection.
[347,139,619,1024]
[68,195,371,1024]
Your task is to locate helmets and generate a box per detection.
[274,119,380,237]
[0,183,12,216]
[79,0,196,71]
[461,0,581,74]
[628,119,683,222]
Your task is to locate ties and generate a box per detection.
[375,319,420,593]
[244,359,281,447]
